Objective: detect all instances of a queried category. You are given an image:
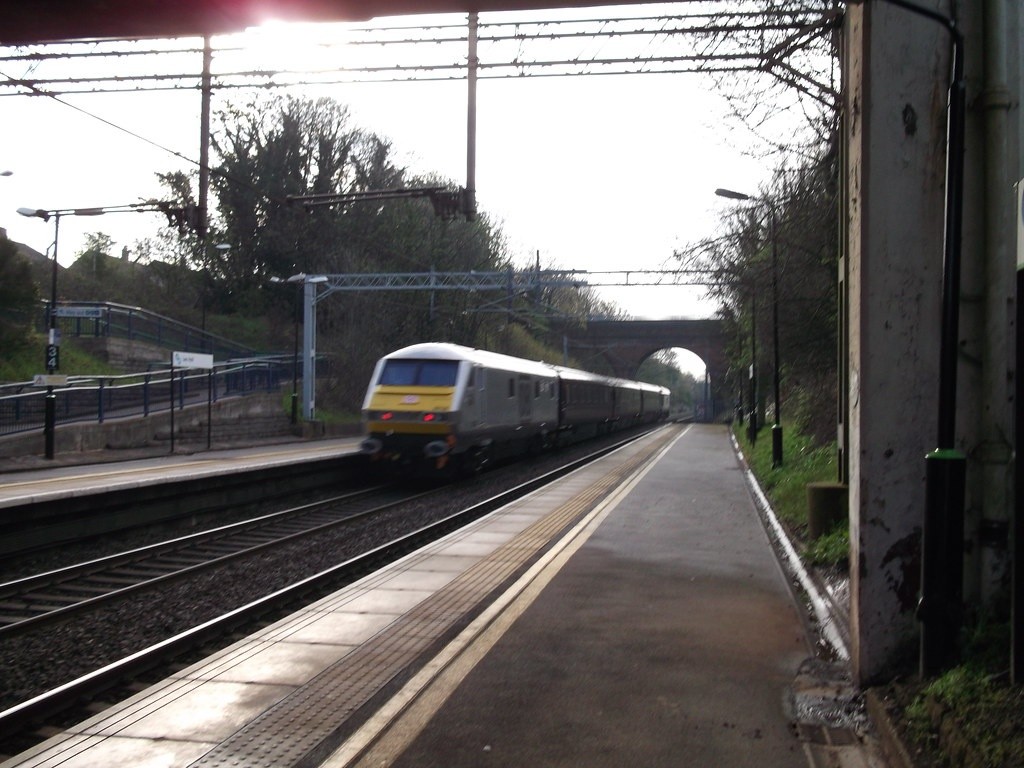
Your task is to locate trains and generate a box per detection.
[362,342,671,479]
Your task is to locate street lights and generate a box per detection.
[216,243,302,424]
[17,208,60,459]
[715,188,783,470]
